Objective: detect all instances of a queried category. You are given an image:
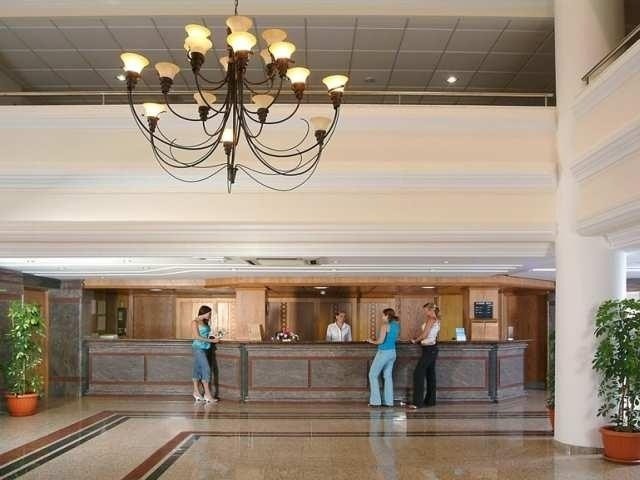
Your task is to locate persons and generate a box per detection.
[366,405,401,480]
[360,308,402,410]
[190,304,222,403]
[193,403,217,480]
[325,312,352,343]
[406,302,441,408]
[404,410,440,480]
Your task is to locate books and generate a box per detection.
[456,328,466,342]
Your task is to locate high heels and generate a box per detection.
[193,394,204,401]
[204,396,218,401]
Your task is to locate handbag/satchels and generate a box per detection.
[420,336,436,346]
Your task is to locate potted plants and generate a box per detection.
[0,299,48,417]
[592,298,640,465]
[545,330,555,432]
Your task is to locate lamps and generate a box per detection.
[116,0,349,193]
